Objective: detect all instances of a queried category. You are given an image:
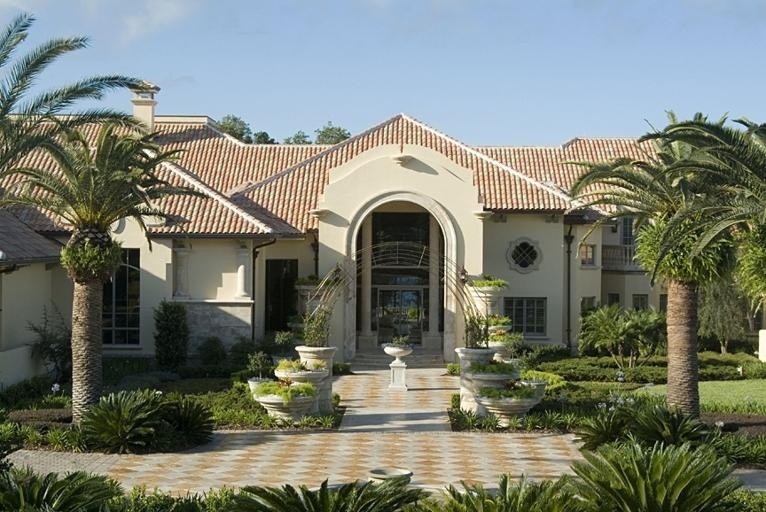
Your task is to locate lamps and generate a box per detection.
[457,264,468,283]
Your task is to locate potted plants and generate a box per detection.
[383,336,414,392]
[246,274,339,424]
[453,273,547,428]
